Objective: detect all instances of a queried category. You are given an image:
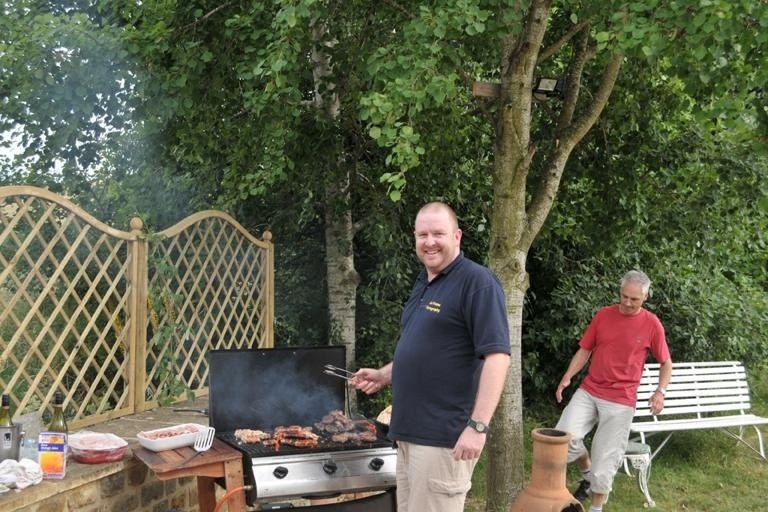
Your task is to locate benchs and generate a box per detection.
[604,358,768,507]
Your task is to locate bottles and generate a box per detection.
[46,390,68,436]
[0,392,14,426]
[507,427,586,512]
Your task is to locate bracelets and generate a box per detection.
[655,386,667,396]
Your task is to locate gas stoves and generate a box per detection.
[213,417,399,505]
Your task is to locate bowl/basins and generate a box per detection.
[68,431,129,464]
[136,422,209,453]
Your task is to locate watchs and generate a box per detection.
[465,417,490,434]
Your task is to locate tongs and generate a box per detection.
[322,365,370,384]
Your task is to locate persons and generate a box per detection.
[553,270,673,512]
[349,201,510,511]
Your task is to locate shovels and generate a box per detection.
[175,426,216,467]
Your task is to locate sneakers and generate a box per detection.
[572,480,590,502]
